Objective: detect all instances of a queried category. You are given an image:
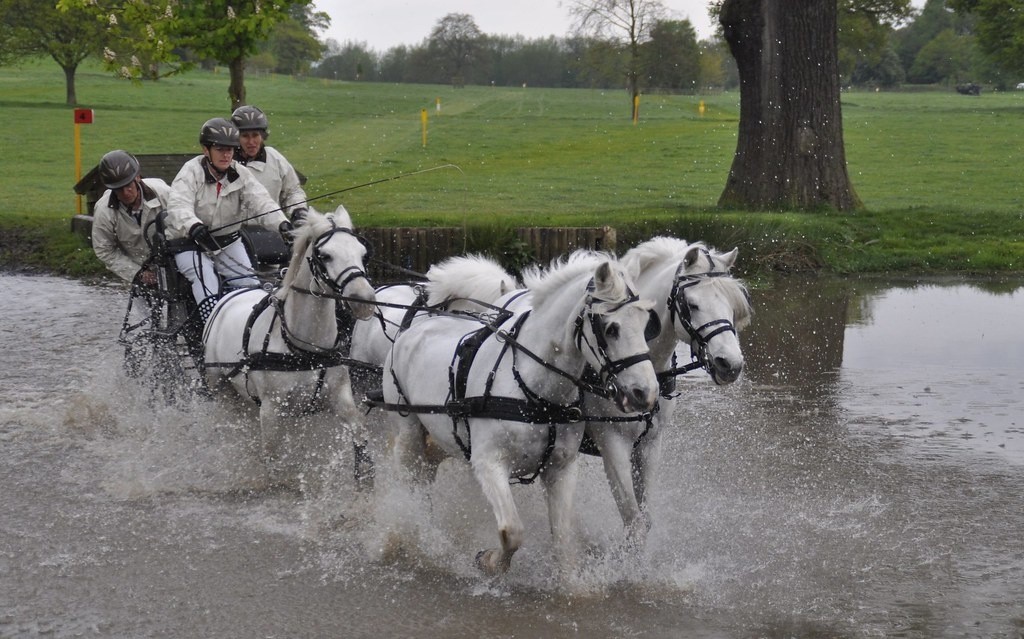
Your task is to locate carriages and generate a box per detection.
[66,150,755,593]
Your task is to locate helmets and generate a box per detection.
[231,106,268,130]
[199,117,240,148]
[98,149,140,189]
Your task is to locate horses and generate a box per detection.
[347,252,518,438]
[201,202,383,491]
[377,249,658,578]
[540,236,745,568]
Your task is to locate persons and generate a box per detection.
[229,105,309,276]
[164,117,291,326]
[91,150,186,294]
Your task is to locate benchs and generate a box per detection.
[129,153,208,188]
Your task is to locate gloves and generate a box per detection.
[292,208,308,227]
[279,220,294,243]
[189,222,210,243]
